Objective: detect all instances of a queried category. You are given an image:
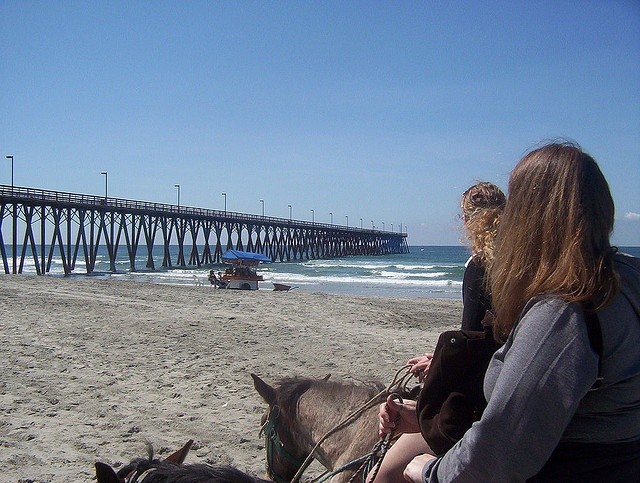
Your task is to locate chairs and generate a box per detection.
[192,274,203,289]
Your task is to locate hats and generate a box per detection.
[419,392,483,456]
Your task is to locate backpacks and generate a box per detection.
[416,289,604,424]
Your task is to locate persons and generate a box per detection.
[363,183,510,483]
[216,271,223,282]
[208,269,224,289]
[378,144,640,482]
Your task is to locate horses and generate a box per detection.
[95,440,268,481]
[251,373,417,483]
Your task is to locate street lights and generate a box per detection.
[405,226,407,235]
[175,184,179,213]
[222,192,226,218]
[100,172,107,205]
[6,156,14,196]
[371,220,374,229]
[288,205,292,223]
[398,224,401,233]
[360,219,363,228]
[382,221,385,231]
[311,209,314,226]
[391,223,393,232]
[330,212,333,228]
[345,216,348,230]
[260,199,264,221]
